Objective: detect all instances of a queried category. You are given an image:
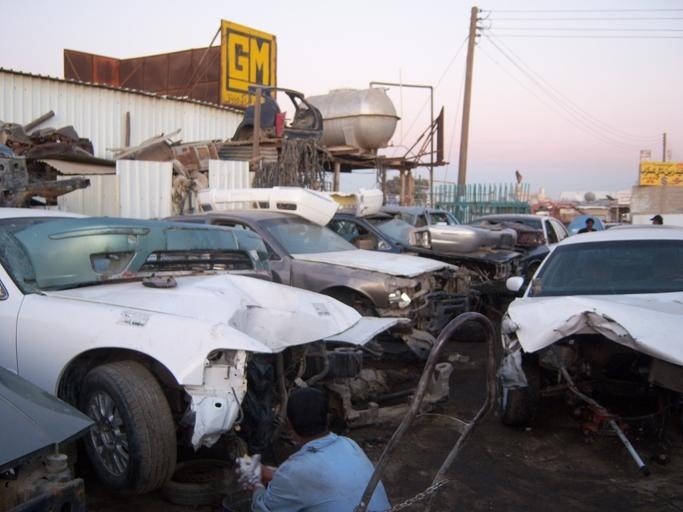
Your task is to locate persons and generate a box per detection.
[233,386,392,511]
[577,217,597,233]
[649,214,663,225]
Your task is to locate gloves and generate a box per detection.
[235,453,264,490]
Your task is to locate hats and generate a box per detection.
[649,214,662,222]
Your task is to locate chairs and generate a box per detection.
[652,253,682,281]
[566,256,617,287]
